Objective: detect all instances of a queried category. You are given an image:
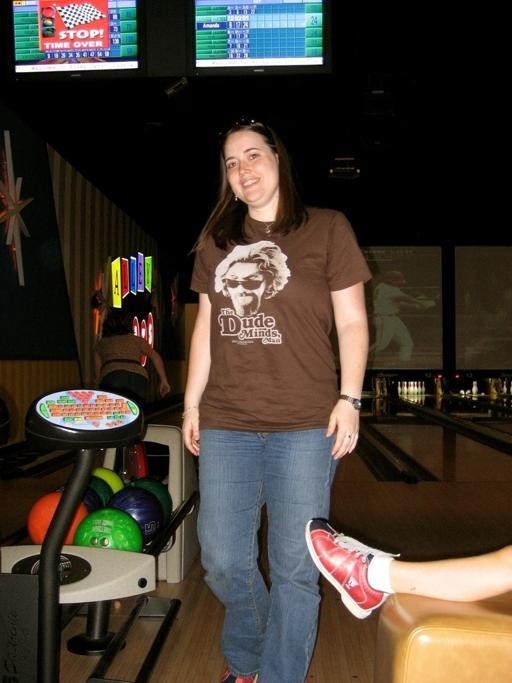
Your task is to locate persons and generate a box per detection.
[367,269,437,364]
[302,516,512,620]
[211,237,293,317]
[178,112,373,682]
[94,310,172,405]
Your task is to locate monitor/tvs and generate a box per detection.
[5,0,147,79]
[187,0,332,76]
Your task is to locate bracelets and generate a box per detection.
[181,404,199,418]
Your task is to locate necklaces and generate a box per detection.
[262,220,274,234]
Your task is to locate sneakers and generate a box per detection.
[305,517,401,619]
[221,668,258,682]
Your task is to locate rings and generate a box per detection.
[345,433,353,438]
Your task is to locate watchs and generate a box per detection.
[339,394,362,410]
